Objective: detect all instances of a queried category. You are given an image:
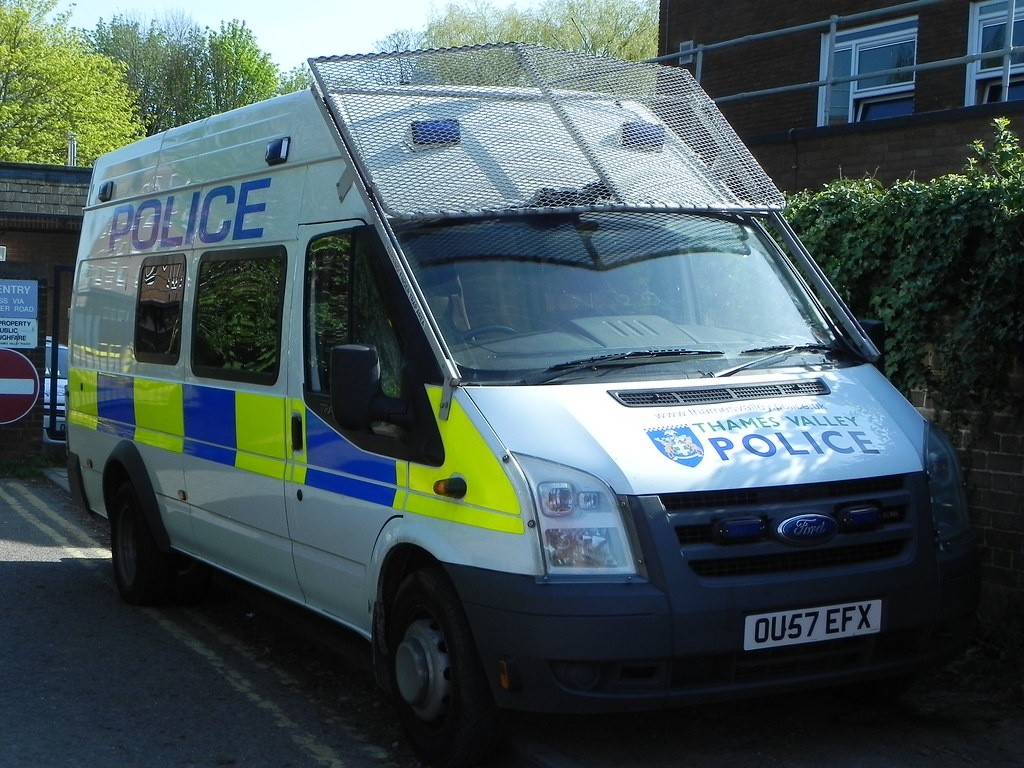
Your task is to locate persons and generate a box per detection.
[360,313,404,397]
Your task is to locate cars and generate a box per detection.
[41,340,71,431]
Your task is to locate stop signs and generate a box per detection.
[0,347,40,425]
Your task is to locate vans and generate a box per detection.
[61,40,988,768]
